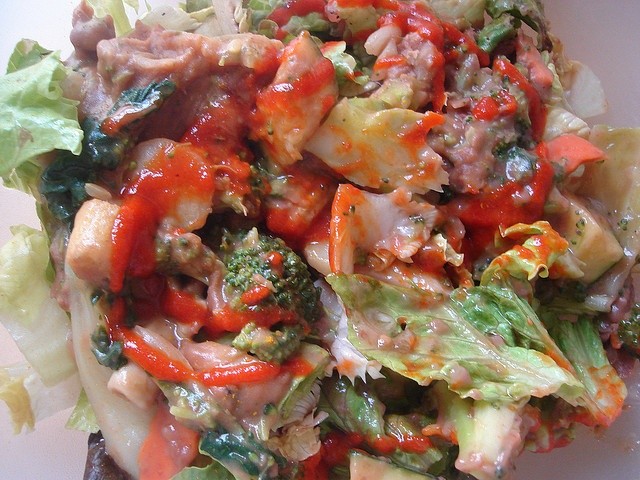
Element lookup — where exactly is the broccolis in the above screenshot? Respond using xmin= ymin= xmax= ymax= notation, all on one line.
xmin=211 ymin=232 xmax=324 ymax=364
